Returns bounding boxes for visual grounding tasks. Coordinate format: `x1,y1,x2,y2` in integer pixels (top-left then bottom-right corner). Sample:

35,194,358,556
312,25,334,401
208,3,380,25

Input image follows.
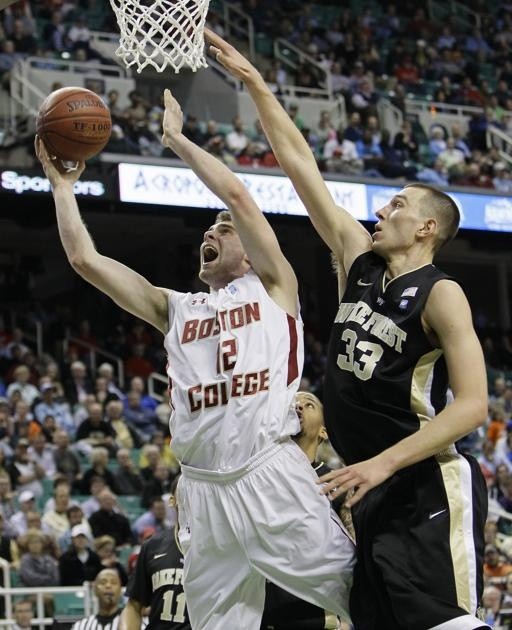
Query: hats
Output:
40,382,57,393
484,542,496,555
73,523,90,540
18,491,34,504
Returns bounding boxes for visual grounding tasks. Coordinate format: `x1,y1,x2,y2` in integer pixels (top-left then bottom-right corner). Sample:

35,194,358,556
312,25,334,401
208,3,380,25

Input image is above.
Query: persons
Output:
0,302,511,628
32,89,360,626
0,0,512,194
204,24,494,629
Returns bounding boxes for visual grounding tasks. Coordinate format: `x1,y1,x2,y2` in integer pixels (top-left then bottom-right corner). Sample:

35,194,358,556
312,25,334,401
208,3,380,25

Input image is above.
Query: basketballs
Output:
37,87,112,160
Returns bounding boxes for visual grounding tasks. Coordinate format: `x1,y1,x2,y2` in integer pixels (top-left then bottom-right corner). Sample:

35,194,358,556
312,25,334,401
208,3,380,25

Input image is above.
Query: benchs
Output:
0,424,155,629
